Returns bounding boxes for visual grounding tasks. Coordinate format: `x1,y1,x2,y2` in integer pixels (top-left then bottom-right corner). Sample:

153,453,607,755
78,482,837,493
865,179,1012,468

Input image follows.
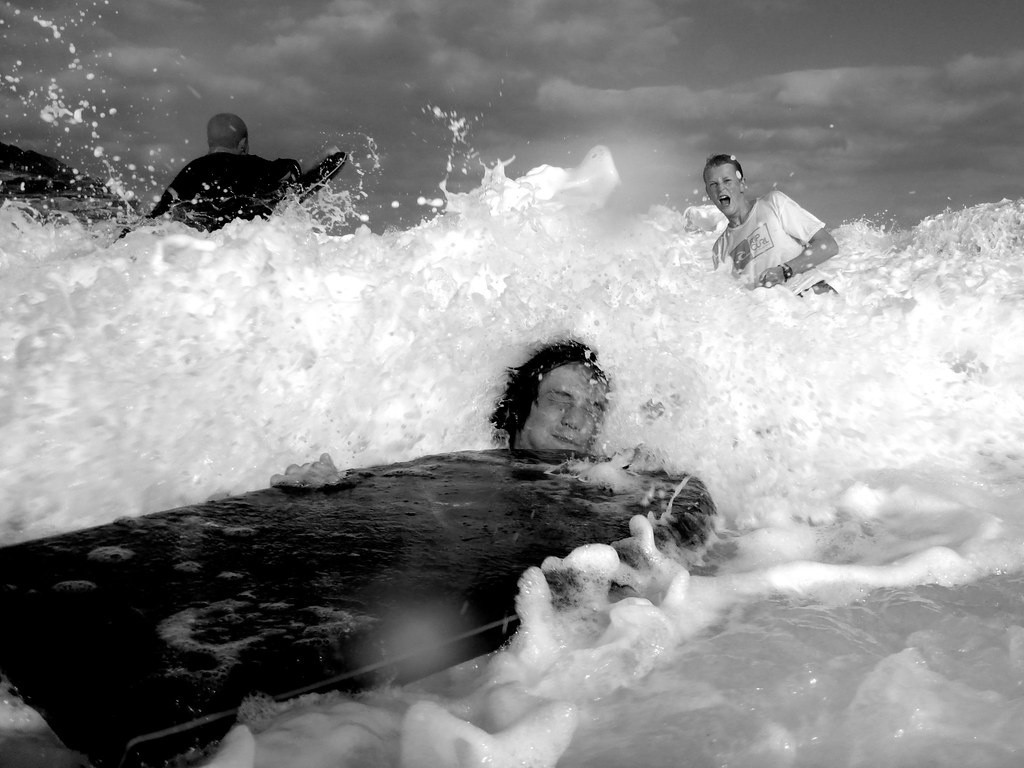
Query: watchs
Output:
779,264,793,281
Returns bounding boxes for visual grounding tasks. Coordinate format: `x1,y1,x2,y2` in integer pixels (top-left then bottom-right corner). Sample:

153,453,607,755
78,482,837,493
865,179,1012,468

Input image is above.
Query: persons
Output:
704,155,839,297
267,338,671,487
118,113,302,241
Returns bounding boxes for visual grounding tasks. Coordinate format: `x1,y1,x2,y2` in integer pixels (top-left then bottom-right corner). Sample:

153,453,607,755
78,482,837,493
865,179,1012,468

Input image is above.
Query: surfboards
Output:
286,151,347,204
0,443,715,768
